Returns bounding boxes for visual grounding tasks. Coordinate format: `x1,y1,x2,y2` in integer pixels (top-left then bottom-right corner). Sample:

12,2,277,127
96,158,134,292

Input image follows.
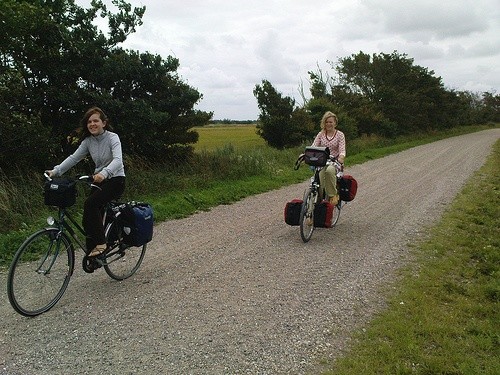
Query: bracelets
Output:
339,157,344,161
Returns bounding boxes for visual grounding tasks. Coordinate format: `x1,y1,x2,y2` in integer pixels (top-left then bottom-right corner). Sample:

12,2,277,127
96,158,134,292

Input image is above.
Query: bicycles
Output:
7,172,149,317
299,154,342,243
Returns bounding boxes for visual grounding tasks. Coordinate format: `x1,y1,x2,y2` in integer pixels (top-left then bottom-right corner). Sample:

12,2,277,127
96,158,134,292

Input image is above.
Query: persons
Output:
44,106,126,258
310,111,347,206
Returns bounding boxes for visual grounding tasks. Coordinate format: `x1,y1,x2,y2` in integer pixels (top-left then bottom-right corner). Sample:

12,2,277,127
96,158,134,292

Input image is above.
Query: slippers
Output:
88,242,109,257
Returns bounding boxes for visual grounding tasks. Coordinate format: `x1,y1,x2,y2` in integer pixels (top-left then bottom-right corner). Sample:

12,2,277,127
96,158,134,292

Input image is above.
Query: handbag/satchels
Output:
124,201,154,245
44,176,77,207
284,199,302,226
339,174,358,202
305,146,329,166
314,201,333,228
